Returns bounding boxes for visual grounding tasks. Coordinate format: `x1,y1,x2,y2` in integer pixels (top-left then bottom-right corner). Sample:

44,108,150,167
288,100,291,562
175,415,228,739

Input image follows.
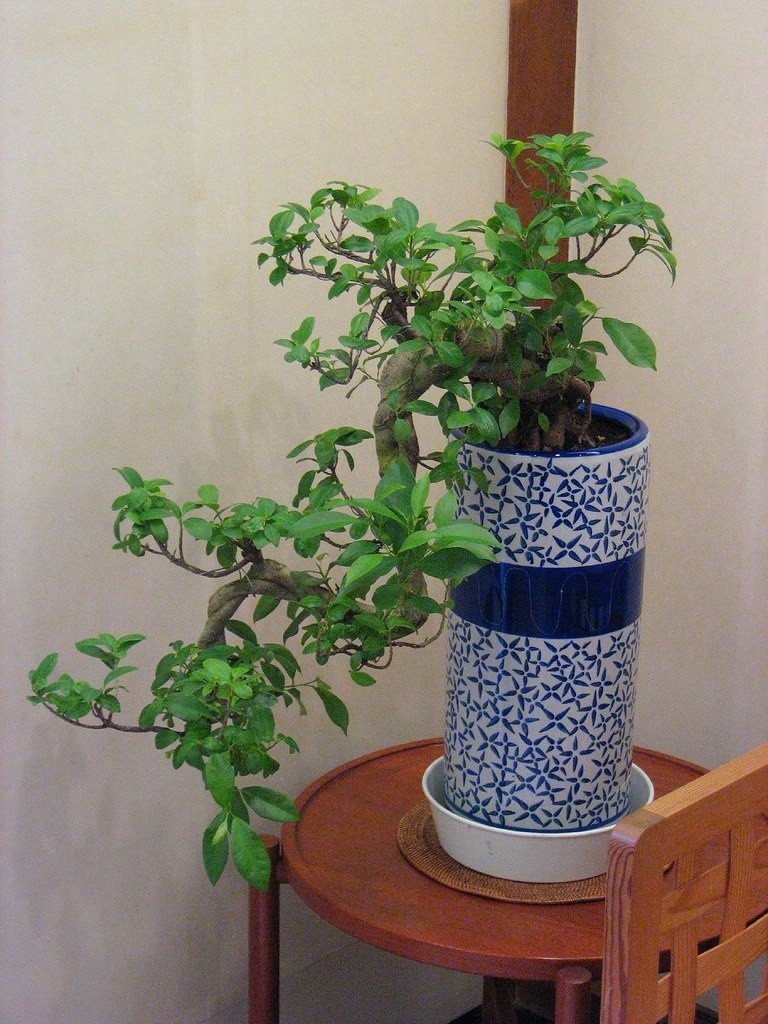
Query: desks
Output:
248,739,713,1024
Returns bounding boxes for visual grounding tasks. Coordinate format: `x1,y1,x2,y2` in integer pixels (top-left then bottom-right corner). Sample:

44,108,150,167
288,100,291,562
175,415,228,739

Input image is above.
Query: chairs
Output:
601,741,764,1023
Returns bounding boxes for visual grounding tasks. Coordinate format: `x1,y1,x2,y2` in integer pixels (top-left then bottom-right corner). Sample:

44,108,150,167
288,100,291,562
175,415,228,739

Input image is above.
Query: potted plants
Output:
24,128,677,893
